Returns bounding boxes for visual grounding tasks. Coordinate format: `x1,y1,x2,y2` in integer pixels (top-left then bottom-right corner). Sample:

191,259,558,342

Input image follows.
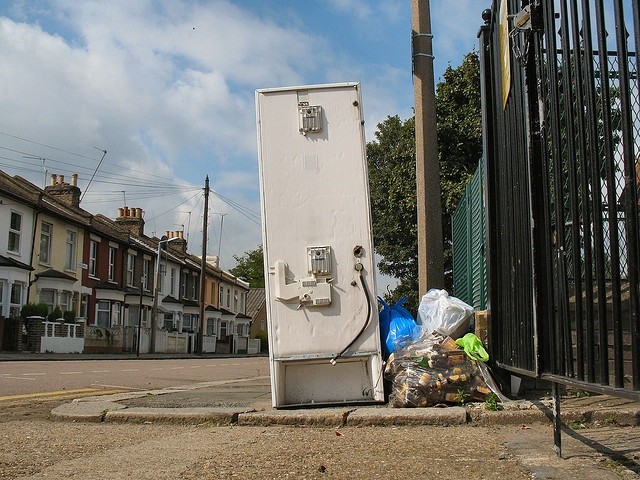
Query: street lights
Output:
152,237,180,301
